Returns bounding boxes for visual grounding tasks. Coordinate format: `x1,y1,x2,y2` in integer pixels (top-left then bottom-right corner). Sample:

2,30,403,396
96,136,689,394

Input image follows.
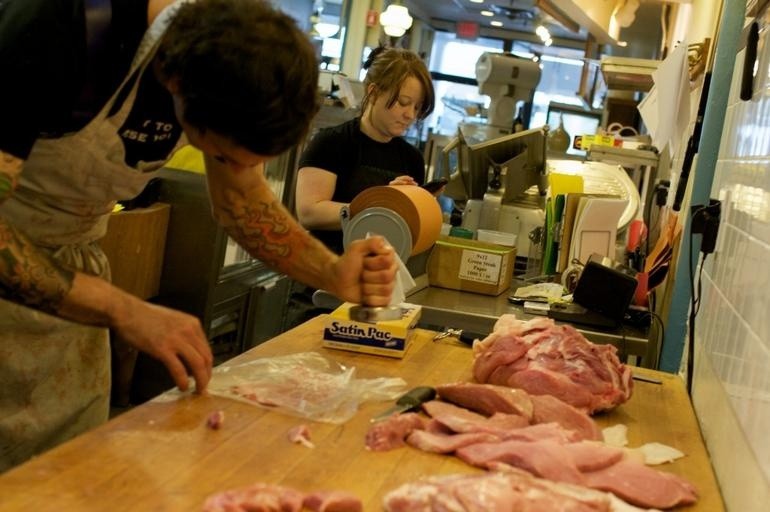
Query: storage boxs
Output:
431,231,518,298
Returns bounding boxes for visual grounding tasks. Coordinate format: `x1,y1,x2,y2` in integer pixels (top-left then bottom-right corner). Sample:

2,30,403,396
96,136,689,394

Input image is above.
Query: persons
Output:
1,1,400,473
293,43,443,328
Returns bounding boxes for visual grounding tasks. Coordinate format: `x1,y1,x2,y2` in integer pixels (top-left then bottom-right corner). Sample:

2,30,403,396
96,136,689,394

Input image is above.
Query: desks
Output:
401,285,653,357
2,312,734,512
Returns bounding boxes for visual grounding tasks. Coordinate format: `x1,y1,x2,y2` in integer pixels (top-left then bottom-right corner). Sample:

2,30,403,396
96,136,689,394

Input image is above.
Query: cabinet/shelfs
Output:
586,55,664,167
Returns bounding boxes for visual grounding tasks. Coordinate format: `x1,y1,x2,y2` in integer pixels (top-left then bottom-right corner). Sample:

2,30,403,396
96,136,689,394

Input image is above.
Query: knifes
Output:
369,385,436,423
